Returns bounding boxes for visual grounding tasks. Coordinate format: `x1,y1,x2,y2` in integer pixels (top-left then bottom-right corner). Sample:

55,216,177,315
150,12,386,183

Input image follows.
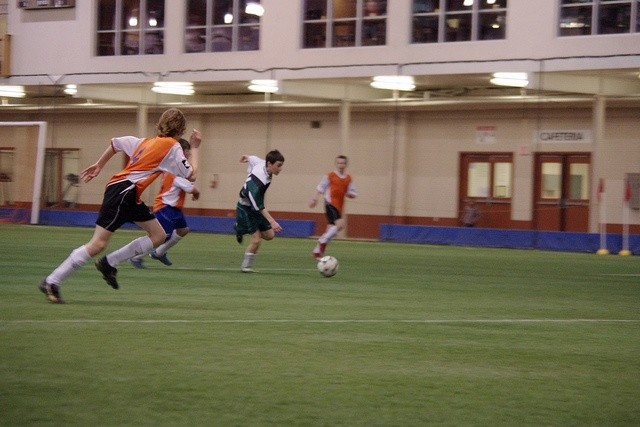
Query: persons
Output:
131,138,199,269
39,109,202,304
309,155,358,261
233,149,284,273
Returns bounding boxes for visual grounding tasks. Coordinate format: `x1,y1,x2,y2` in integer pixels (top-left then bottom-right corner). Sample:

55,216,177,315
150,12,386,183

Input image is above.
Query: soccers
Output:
317,256,339,277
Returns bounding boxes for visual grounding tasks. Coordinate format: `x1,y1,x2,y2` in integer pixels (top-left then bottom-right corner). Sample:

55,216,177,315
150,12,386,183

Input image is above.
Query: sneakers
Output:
128,258,147,269
240,267,260,273
233,222,242,243
94,255,119,289
149,250,172,265
38,278,62,303
312,250,320,260
318,239,327,256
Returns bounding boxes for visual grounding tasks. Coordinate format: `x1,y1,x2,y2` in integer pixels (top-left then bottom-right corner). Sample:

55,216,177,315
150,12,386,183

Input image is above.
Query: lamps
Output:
0,84,77,99
370,72,530,92
152,79,278,96
